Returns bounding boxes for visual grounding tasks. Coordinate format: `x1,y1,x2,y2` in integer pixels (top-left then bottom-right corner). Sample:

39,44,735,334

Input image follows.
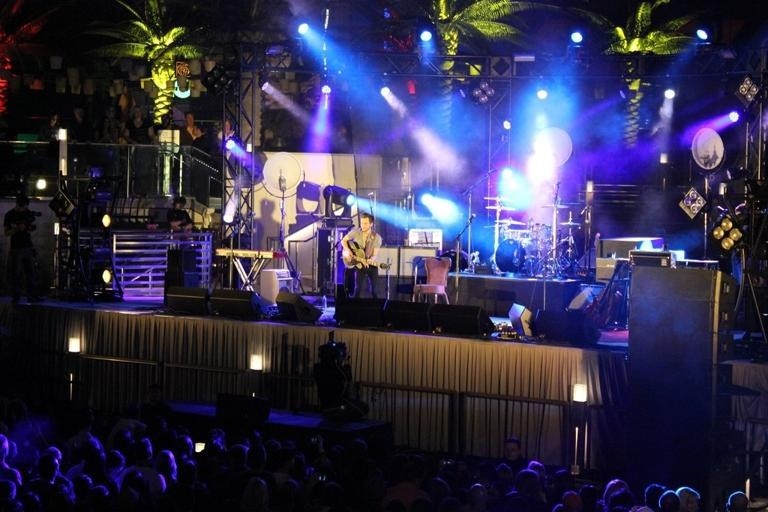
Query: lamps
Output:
573,383,588,425
323,184,353,227
249,353,264,391
67,338,81,368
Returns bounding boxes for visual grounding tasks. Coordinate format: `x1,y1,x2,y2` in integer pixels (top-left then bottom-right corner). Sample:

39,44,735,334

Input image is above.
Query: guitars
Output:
586,259,628,331
342,240,391,270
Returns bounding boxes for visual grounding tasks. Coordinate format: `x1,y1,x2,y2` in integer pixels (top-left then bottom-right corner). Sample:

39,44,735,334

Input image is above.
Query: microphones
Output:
467,213,478,224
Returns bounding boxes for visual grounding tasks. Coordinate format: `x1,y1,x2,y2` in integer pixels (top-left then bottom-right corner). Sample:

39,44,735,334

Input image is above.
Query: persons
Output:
3,192,45,306
0,420,764,512
167,196,193,231
49,67,372,204
341,214,382,299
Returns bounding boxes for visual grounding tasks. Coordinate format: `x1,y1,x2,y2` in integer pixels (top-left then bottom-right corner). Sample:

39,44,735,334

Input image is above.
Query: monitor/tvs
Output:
148,207,171,224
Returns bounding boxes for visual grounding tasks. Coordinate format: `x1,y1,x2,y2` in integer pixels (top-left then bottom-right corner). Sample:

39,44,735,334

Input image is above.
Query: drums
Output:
496,238,534,273
508,230,531,250
534,224,551,250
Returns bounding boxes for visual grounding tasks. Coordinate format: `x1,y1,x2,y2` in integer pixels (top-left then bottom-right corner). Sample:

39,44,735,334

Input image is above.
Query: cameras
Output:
13,210,42,226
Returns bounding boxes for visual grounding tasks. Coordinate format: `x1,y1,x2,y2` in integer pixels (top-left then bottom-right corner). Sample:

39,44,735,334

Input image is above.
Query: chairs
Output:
411,255,453,305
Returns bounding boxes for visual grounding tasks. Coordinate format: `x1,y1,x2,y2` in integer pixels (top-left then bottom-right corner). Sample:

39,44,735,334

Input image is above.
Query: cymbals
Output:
486,206,514,211
561,222,580,226
483,196,511,201
542,205,569,209
495,219,526,226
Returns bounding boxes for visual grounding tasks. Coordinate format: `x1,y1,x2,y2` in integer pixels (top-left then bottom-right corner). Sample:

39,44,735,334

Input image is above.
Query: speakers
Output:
274,290,323,323
335,297,496,339
531,308,603,346
622,265,739,511
165,286,268,321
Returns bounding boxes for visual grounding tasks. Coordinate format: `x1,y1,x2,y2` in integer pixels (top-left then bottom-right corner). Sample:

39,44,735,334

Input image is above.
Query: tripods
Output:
260,176,306,295
